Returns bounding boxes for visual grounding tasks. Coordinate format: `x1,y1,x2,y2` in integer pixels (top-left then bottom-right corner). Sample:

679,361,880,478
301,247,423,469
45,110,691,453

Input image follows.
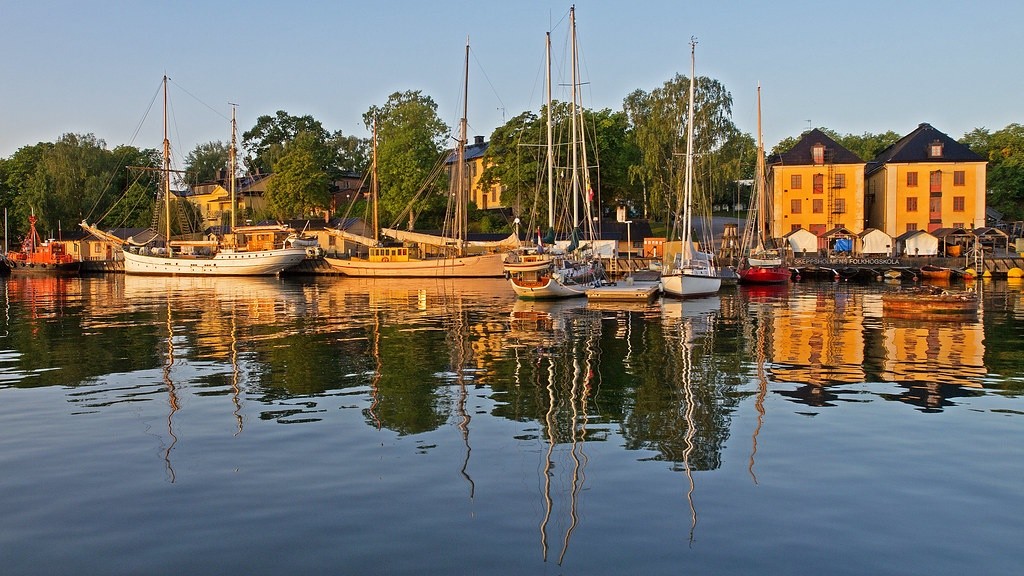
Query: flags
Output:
585,177,594,201
538,228,544,253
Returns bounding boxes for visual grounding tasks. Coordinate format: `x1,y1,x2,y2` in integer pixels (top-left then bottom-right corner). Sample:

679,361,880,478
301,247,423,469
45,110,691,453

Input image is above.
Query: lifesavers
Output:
3,259,46,268
381,257,389,262
306,246,319,258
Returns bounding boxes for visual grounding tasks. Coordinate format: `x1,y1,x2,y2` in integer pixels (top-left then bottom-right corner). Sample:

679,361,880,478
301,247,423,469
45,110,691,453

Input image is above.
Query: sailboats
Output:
76,4,790,304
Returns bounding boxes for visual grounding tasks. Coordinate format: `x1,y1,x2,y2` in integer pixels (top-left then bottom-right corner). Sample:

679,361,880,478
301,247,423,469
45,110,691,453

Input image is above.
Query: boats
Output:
7,207,79,268
920,265,951,279
